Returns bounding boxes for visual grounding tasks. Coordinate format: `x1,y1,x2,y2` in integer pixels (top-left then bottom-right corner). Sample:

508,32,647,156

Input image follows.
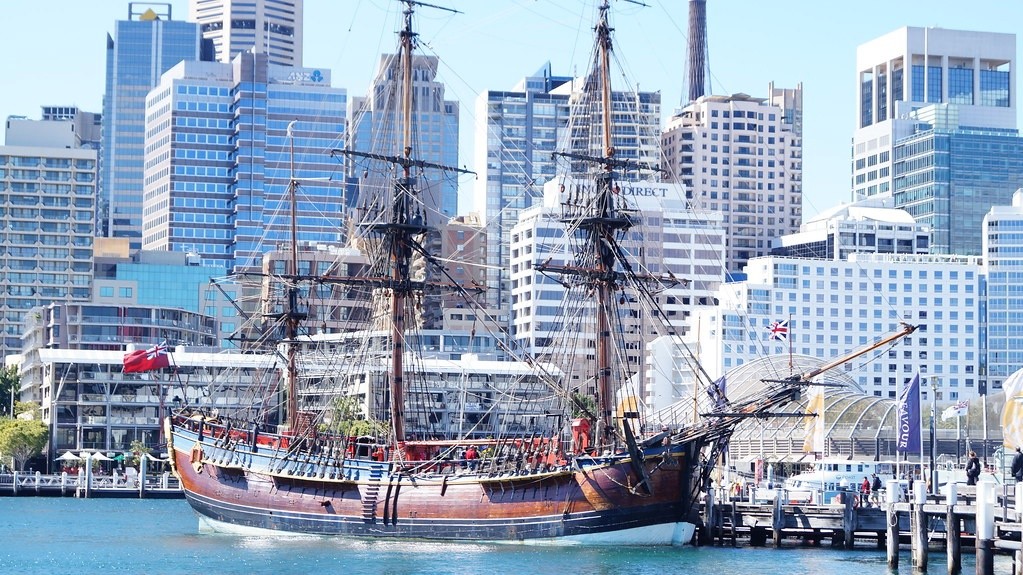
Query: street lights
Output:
923,374,947,492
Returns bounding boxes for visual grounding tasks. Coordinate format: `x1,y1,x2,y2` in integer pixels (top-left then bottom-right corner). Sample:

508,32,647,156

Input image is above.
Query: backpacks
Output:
967,462,980,477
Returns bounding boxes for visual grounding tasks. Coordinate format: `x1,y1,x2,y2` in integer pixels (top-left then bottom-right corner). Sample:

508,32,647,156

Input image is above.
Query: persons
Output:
170,412,698,484
861,471,919,509
716,474,788,506
0,464,15,482
1011,446,1023,482
63,461,172,477
963,450,981,504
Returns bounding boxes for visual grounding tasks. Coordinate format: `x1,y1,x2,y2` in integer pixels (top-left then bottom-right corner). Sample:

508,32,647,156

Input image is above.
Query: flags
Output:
123,341,170,374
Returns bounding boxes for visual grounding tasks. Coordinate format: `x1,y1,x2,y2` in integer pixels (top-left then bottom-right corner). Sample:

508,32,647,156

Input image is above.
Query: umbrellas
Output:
90,452,114,461
53,451,82,466
766,317,791,342
146,452,165,463
111,454,127,463
941,399,969,422
794,469,870,507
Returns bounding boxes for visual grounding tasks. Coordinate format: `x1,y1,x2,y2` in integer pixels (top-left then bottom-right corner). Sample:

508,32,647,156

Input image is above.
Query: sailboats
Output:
160,0,919,554
